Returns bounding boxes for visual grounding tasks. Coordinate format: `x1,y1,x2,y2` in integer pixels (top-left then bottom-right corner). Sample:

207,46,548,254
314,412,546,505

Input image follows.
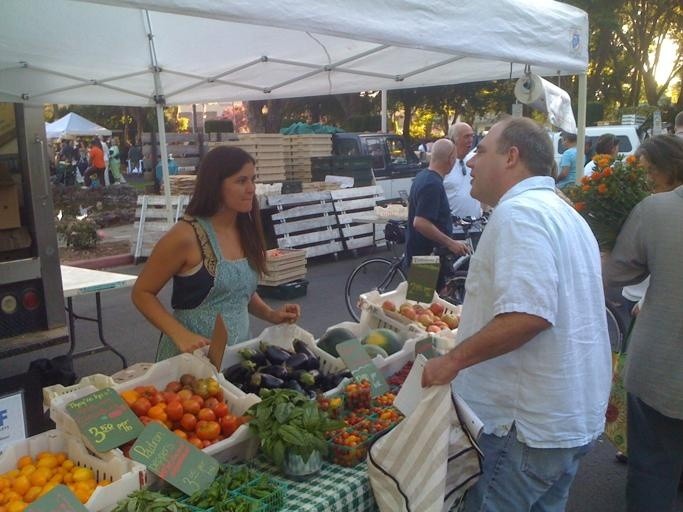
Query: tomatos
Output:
121,374,256,458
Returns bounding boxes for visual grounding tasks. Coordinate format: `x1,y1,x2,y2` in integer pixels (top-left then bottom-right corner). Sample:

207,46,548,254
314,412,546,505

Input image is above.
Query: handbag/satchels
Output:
366,383,484,512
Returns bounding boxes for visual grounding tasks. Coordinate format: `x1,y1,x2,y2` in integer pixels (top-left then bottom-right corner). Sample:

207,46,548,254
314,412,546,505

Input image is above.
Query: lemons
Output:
0,451,112,512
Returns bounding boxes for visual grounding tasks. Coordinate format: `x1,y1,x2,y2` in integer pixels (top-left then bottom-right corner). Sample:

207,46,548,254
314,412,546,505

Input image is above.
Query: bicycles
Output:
345,214,489,323
603,303,625,356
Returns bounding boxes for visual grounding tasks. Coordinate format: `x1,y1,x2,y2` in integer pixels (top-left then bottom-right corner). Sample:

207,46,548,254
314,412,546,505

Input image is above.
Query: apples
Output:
382,299,460,333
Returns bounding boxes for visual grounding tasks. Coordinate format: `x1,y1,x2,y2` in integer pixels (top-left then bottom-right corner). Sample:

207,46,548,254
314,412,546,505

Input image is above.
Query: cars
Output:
331,134,427,207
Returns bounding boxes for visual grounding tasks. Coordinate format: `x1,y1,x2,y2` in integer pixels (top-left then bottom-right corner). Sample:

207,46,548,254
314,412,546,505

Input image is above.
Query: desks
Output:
244,434,380,512
59,262,140,377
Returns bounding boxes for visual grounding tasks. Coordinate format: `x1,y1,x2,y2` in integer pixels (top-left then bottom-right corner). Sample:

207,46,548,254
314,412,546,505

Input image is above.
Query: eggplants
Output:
222,339,352,399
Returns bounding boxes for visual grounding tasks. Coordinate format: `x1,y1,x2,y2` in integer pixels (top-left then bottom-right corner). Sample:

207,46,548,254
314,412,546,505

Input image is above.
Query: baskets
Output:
315,321,423,380
48,352,256,487
359,281,462,339
320,403,405,468
0,428,147,512
151,462,288,512
193,322,355,403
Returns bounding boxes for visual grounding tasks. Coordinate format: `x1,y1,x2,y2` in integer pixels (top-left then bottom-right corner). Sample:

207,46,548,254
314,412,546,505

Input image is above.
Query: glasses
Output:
460,160,466,175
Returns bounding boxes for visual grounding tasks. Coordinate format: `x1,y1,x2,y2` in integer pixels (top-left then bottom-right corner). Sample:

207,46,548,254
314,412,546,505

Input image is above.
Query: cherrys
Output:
316,379,404,467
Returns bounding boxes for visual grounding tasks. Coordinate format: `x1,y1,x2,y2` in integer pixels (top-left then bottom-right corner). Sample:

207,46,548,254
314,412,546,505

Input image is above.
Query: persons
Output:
674,111,683,139
405,137,470,298
130,145,302,363
555,130,586,197
583,134,621,181
442,122,491,302
47,133,142,191
585,135,594,167
634,132,682,192
420,116,613,512
604,184,683,512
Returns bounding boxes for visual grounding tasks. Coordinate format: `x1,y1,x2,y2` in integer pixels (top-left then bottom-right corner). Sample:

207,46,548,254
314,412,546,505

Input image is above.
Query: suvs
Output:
547,124,641,173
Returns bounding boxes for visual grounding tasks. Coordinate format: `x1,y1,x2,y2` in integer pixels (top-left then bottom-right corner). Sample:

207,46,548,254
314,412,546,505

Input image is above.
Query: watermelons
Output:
316,328,404,359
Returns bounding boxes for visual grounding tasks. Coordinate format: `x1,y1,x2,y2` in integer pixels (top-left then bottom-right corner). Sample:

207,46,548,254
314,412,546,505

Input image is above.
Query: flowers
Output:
561,148,652,232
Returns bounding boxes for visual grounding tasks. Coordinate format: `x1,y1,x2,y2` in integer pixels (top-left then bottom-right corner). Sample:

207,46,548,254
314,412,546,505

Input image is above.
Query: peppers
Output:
186,463,281,512
